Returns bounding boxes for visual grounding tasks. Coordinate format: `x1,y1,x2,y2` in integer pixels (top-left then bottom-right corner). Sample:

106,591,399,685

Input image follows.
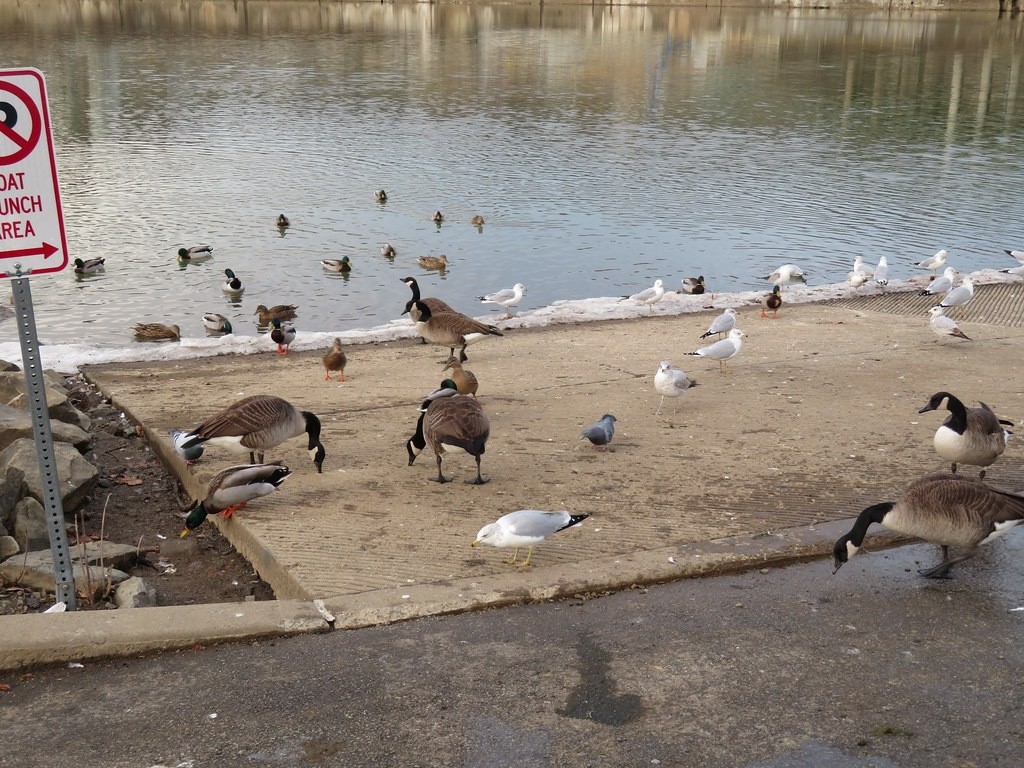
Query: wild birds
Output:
763,263,807,287
919,391,1014,481
910,248,1024,315
654,360,701,416
407,394,490,486
681,275,705,294
72,189,484,381
831,473,1023,578
475,282,528,318
846,255,890,293
682,329,749,374
180,459,294,539
472,510,593,569
926,306,973,345
180,394,327,474
758,284,782,319
700,307,740,342
399,277,504,364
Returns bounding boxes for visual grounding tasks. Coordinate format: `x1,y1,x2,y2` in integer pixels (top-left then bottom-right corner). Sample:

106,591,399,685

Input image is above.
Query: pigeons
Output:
167,428,208,466
580,414,617,452
